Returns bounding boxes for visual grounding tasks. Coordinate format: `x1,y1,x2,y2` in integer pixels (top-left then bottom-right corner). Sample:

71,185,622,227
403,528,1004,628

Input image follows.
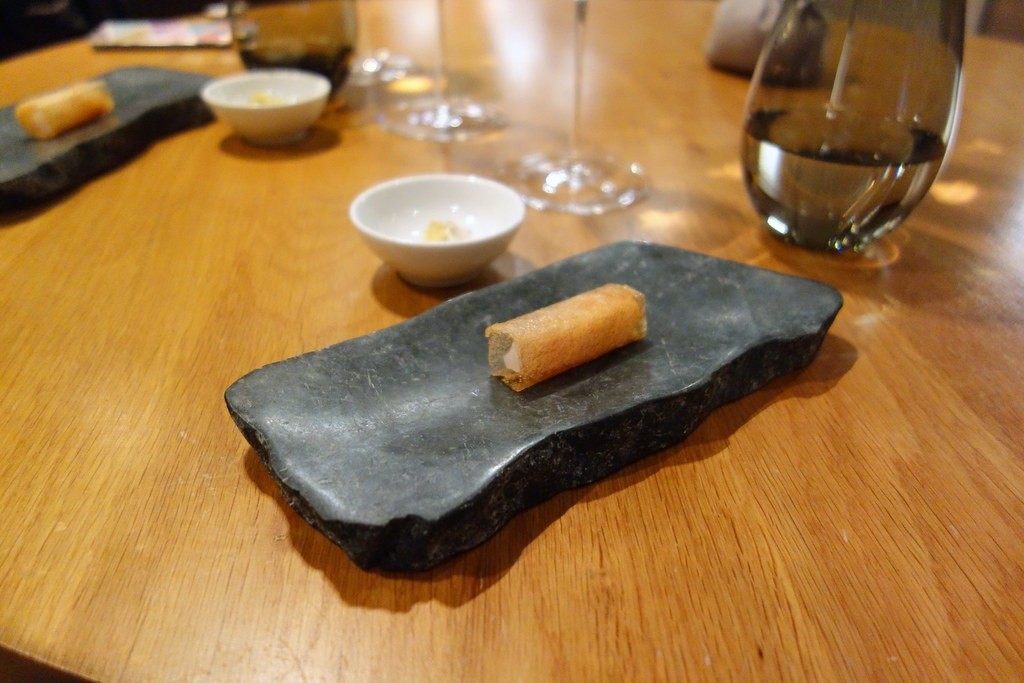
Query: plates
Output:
221,237,848,578
0,64,220,214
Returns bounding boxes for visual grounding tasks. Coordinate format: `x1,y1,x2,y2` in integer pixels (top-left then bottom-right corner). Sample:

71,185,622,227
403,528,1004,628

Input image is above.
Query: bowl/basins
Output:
353,174,528,292
202,71,331,148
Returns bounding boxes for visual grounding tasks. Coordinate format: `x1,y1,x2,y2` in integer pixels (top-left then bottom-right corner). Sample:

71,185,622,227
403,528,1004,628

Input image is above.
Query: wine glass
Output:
357,2,651,222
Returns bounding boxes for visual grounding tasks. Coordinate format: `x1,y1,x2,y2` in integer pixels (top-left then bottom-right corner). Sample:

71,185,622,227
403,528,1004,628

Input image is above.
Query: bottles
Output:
223,1,355,98
734,0,971,253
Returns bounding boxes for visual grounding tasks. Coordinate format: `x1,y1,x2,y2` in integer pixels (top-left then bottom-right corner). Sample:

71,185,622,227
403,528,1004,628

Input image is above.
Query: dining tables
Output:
0,0,1024,682
